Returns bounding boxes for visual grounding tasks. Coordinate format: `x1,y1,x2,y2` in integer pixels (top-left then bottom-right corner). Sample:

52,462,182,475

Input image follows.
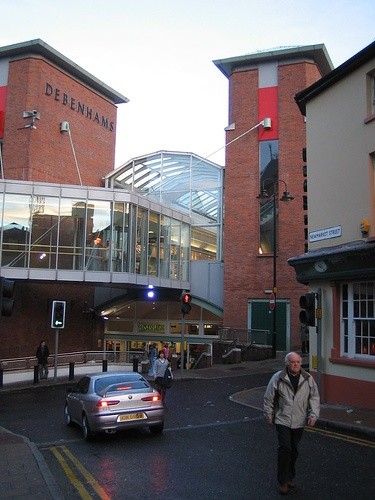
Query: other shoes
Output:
288,481,296,489
279,483,289,493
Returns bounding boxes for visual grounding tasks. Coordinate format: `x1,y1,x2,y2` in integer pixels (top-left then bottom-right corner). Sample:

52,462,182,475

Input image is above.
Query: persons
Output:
152,351,171,404
151,343,159,363
164,345,170,360
262,351,320,494
36,341,50,380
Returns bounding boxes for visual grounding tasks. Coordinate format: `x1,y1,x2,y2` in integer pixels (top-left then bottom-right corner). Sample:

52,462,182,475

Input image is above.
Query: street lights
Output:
256,178,294,359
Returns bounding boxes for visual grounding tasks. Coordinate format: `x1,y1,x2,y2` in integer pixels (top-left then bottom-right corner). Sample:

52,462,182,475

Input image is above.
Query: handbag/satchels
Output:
164,363,171,380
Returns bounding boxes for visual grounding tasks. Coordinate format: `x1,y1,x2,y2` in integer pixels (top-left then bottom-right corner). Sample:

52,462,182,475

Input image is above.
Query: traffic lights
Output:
180,293,192,313
51,301,65,329
298,292,316,327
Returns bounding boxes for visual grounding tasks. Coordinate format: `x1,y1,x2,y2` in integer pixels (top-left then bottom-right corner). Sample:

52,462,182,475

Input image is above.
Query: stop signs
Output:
269,293,276,310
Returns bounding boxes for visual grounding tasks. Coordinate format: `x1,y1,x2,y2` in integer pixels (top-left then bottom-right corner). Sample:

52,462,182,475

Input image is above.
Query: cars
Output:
63,372,165,445
